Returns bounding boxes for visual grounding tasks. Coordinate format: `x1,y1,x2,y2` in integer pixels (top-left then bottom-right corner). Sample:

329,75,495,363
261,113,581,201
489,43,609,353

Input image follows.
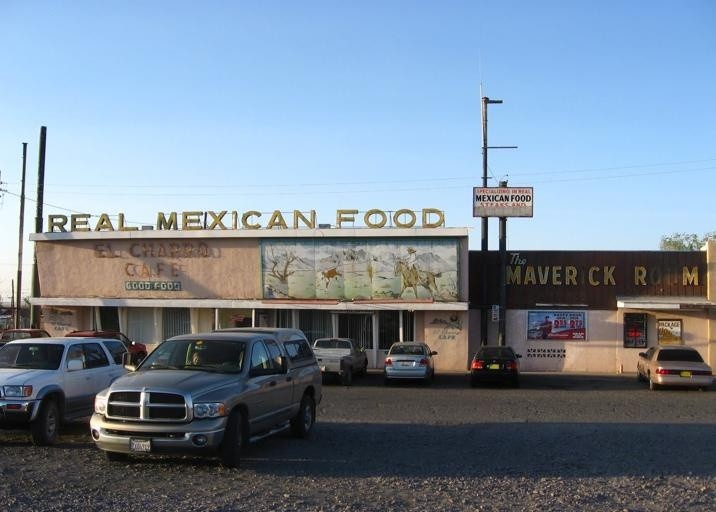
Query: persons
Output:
539,317,553,339
191,353,202,367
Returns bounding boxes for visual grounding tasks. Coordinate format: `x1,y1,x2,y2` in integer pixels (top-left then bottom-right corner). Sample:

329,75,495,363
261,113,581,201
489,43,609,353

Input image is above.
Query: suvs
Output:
0,335,148,448
66,329,148,367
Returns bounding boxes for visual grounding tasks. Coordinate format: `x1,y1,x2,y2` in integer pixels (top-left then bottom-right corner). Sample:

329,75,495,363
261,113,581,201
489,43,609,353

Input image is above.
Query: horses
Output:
393,259,442,303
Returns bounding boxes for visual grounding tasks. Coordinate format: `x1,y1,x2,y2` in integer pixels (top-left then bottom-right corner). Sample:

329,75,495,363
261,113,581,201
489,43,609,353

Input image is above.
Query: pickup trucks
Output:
310,336,369,385
88,323,324,470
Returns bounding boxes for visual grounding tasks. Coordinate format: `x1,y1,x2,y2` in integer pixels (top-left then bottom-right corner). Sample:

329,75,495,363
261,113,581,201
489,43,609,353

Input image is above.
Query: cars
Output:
469,342,524,390
380,340,440,387
0,326,52,352
634,345,715,391
